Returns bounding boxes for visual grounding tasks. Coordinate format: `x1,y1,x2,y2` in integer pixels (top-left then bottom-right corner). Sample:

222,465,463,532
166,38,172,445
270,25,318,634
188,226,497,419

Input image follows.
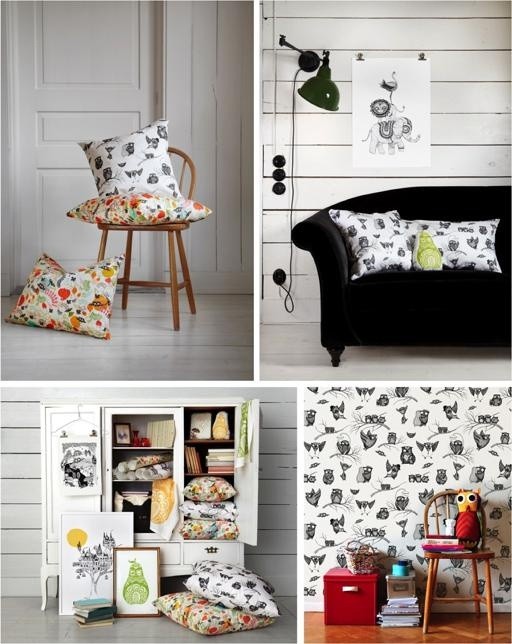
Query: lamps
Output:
276,31,340,112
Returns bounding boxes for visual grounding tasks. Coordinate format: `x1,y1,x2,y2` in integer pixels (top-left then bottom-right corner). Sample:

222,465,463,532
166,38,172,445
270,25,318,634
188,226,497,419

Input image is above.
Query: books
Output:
183,445,235,474
422,534,472,555
377,596,422,627
73,598,118,628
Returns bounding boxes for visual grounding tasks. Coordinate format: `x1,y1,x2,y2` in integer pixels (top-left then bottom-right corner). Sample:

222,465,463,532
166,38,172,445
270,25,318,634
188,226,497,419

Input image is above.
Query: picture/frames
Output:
59,511,165,617
114,421,132,447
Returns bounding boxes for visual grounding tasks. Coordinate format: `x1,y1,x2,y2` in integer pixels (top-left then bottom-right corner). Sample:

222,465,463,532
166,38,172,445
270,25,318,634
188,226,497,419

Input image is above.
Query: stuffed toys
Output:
452,488,482,550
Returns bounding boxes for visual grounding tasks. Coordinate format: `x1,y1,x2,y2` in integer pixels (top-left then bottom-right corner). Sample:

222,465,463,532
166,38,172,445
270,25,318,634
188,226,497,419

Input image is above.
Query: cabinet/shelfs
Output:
101,397,259,610
39,397,104,612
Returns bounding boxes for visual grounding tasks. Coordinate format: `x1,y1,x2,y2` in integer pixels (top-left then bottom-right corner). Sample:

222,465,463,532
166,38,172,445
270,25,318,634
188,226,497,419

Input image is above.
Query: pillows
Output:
178,475,240,539
66,117,214,225
325,205,503,279
5,252,126,339
154,559,281,634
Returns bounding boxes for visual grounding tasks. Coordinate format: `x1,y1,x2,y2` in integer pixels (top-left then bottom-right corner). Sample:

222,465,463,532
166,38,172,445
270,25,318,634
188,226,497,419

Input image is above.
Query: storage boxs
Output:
323,565,416,624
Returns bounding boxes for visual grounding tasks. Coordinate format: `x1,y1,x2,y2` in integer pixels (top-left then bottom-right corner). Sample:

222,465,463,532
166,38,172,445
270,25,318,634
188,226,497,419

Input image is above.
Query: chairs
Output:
422,489,495,634
97,147,197,330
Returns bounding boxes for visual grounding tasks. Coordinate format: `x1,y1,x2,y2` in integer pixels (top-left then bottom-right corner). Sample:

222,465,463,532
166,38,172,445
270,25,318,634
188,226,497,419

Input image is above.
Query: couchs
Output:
291,185,510,366
